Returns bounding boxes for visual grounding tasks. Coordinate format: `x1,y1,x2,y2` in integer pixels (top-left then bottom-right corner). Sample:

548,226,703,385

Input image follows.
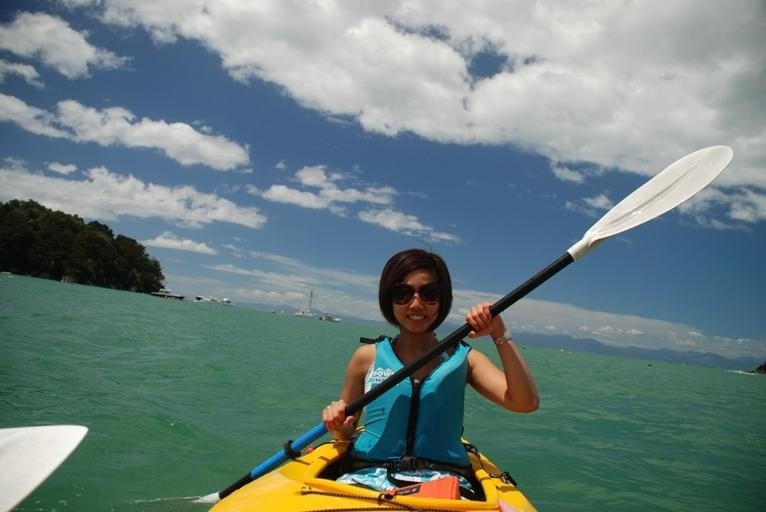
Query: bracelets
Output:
493,333,512,345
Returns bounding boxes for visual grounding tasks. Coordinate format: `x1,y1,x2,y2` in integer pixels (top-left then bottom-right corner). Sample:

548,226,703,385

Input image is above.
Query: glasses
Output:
391,283,441,304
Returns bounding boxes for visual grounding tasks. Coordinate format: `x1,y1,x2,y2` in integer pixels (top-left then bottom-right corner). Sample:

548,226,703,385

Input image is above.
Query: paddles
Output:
193,145,734,505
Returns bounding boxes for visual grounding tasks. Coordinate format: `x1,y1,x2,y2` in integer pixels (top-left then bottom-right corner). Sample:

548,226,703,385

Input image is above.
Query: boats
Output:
206,431,536,512
1,423,90,512
148,284,341,325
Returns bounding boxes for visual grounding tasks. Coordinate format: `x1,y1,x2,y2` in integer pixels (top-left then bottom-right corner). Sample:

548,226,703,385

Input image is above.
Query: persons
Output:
321,249,539,503
